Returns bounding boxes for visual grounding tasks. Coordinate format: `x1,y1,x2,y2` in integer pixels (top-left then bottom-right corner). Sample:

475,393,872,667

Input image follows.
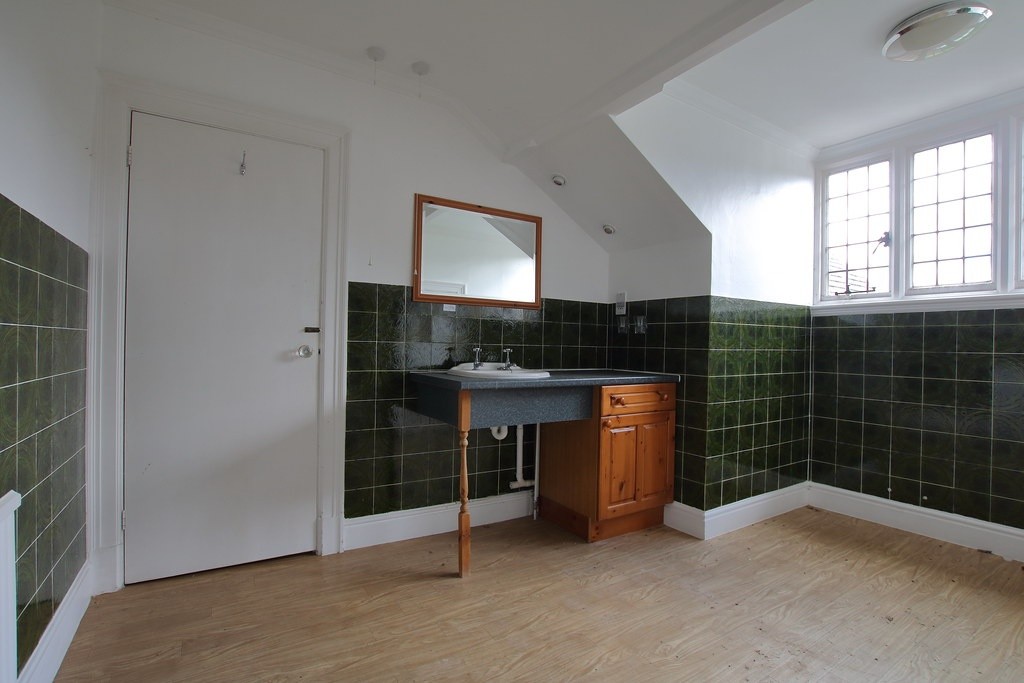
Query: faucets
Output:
472,348,485,370
498,349,516,370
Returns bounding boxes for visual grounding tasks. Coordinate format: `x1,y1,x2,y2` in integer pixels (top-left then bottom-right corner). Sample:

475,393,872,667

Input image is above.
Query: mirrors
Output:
412,193,541,310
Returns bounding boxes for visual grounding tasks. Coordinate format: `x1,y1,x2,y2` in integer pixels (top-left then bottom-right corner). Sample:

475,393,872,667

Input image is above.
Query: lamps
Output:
881,0,993,63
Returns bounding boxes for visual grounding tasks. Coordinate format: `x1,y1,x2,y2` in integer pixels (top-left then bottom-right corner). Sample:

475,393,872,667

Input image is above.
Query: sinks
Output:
447,360,550,380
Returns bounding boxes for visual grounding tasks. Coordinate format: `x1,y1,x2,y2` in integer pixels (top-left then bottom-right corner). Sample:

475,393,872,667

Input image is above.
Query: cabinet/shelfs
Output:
538,383,677,544
